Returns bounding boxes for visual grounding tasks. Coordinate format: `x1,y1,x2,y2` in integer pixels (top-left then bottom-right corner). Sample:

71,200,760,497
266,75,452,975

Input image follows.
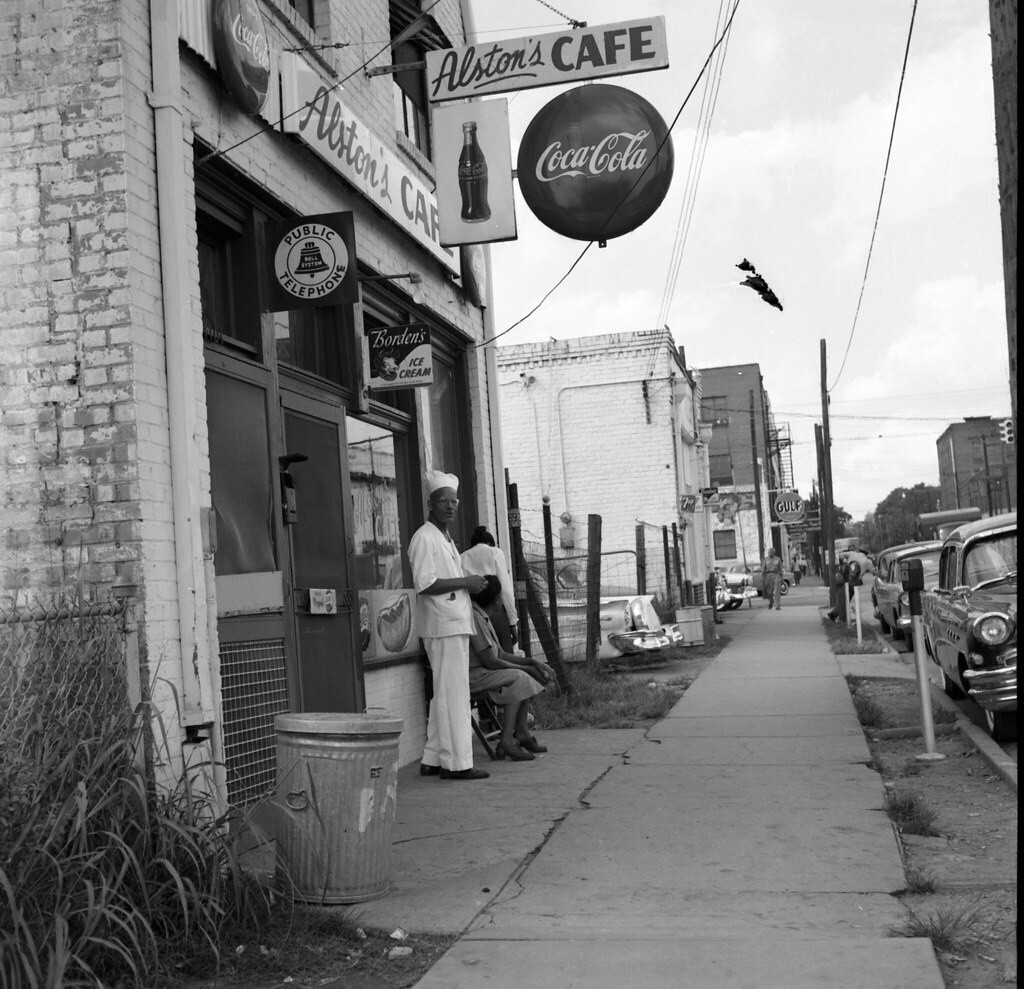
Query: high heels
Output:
519,737,548,753
495,741,535,760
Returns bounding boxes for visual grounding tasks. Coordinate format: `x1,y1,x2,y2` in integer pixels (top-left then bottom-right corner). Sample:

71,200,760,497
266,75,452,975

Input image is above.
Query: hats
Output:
424,469,459,496
467,573,503,607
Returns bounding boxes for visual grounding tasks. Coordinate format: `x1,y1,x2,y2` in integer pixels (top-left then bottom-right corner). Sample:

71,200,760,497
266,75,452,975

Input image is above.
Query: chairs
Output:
425,668,504,760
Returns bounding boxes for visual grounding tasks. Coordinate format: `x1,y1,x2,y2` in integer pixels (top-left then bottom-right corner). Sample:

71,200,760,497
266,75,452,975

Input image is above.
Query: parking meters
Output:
840,561,853,630
896,555,945,765
849,559,864,647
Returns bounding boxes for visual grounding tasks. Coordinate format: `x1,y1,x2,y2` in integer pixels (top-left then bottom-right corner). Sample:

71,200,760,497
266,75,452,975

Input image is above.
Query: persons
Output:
406,470,490,779
468,575,556,761
801,558,807,575
828,545,886,624
761,548,783,610
459,526,518,741
792,557,801,586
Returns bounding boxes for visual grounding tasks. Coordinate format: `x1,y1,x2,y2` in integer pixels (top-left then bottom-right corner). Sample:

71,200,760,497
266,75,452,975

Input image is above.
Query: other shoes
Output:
438,767,490,780
827,611,837,621
776,606,781,610
420,763,439,776
768,601,774,609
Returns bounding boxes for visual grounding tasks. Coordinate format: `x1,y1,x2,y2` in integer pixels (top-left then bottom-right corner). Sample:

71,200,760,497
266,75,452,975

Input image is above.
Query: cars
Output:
523,552,661,612
726,562,796,596
919,511,1018,739
869,539,943,652
526,577,683,663
714,567,754,612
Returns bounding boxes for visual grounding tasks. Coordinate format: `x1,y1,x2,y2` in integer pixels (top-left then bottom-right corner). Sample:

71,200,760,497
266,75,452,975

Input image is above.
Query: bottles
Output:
457,121,492,224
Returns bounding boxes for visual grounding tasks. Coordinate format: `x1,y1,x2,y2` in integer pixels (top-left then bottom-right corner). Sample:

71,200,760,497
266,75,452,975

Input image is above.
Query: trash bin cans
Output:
274,707,404,904
